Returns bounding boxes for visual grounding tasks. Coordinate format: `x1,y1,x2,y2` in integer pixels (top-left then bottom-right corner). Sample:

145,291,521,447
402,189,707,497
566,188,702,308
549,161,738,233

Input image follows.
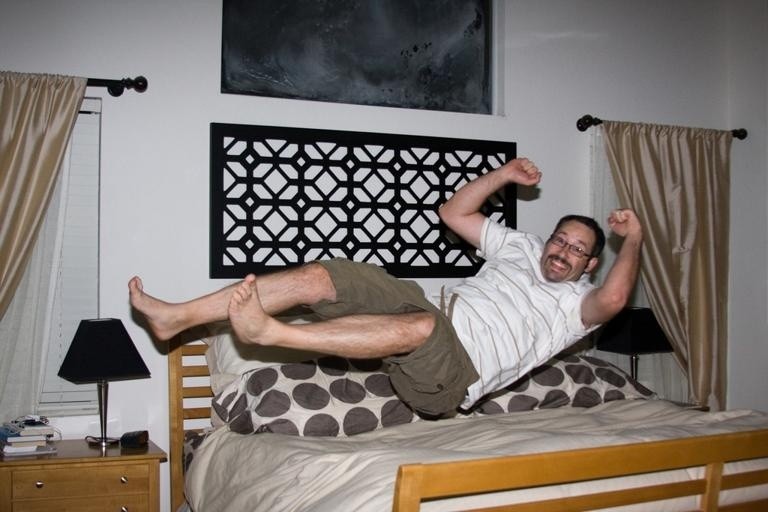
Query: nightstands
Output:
661,396,712,414
1,436,169,511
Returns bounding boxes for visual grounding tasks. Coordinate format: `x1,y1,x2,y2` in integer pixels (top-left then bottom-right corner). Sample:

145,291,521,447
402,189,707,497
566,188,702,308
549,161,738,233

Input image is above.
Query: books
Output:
0,421,59,457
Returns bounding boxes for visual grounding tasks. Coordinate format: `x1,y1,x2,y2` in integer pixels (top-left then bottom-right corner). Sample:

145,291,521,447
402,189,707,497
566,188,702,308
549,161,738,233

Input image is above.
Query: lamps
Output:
594,305,677,384
55,316,149,451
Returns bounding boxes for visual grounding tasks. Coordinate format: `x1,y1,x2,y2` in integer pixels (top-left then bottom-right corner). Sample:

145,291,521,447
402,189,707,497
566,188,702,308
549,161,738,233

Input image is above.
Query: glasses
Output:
551,234,591,257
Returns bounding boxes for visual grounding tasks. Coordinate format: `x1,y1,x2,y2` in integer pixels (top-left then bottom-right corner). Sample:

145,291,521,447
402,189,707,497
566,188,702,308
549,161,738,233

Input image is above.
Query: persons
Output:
127,157,645,420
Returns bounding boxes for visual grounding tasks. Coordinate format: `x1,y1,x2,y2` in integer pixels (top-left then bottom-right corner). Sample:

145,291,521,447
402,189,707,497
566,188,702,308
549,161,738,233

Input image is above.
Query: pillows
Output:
196,309,321,429
440,353,658,420
209,354,429,438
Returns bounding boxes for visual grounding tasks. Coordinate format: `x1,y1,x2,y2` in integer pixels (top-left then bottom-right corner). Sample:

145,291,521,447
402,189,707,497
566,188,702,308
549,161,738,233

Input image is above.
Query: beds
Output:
164,302,768,510
